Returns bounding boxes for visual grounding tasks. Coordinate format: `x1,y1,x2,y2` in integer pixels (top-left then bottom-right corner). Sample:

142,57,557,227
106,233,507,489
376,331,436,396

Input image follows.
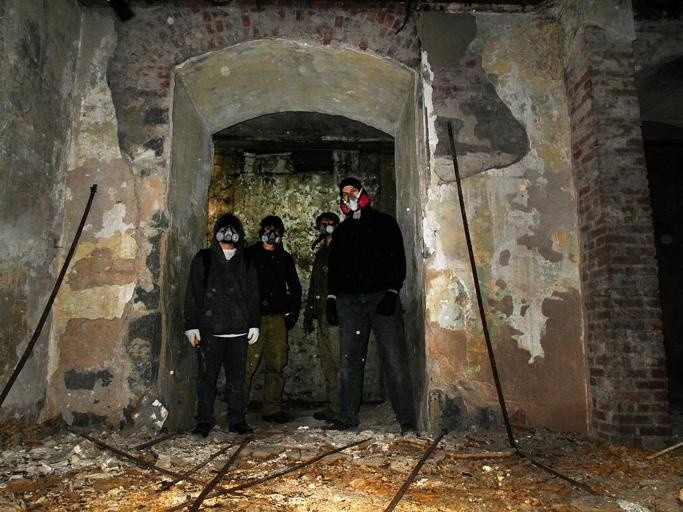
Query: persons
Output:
321,176,423,438
303,212,343,423
243,214,303,425
182,211,264,440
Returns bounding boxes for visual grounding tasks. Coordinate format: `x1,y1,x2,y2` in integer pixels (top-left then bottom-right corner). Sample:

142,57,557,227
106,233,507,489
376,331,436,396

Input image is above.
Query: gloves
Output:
286,313,299,330
184,328,202,348
303,317,316,333
247,328,260,345
376,291,398,316
325,298,339,326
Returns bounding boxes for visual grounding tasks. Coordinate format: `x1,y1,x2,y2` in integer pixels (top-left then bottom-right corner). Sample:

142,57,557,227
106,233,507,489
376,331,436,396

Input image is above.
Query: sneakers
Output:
191,407,420,439
